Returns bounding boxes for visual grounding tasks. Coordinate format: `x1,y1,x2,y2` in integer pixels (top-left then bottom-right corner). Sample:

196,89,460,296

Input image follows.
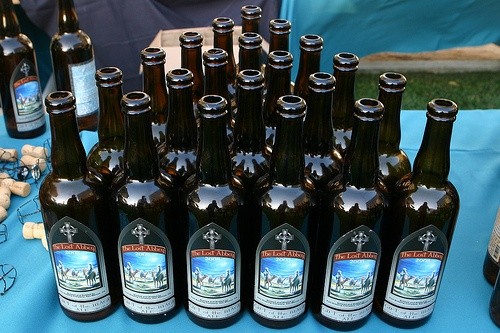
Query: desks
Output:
0,110,500,333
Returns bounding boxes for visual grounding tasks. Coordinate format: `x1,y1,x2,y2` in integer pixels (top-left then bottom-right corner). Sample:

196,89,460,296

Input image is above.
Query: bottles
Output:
482,205,500,287
488,269,500,330
0,0,461,331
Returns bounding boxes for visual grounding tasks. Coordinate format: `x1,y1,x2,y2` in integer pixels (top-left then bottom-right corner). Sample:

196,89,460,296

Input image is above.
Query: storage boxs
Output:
139,26,269,75
